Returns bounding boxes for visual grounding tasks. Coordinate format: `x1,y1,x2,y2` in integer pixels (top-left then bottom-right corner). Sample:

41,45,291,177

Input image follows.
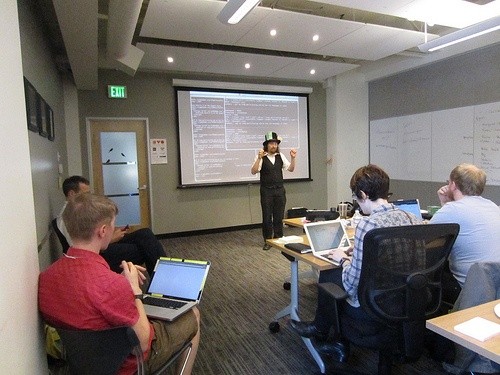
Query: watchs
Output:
340,258,349,265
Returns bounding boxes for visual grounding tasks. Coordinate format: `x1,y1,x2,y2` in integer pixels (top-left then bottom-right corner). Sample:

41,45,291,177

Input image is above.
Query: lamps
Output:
217,0,500,55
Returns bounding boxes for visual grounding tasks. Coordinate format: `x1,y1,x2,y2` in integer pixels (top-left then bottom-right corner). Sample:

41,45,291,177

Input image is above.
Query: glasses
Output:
352,193,360,201
446,178,453,184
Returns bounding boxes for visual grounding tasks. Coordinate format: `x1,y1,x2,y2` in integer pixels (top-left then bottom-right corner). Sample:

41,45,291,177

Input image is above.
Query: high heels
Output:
289,318,328,343
311,336,348,363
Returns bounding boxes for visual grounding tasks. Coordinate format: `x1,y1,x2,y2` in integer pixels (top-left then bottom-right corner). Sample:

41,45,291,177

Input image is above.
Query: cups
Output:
427,206,441,215
339,204,347,219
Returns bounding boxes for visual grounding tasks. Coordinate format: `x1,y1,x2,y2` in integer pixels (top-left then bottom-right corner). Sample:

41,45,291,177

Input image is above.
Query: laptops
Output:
304,220,353,266
392,199,422,221
141,257,210,322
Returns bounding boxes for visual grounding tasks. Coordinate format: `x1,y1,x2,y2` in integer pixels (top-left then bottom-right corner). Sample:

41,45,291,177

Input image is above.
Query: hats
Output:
263,131,281,146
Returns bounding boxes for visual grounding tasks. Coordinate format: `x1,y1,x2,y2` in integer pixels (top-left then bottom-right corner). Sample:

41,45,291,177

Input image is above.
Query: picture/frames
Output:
23,76,55,140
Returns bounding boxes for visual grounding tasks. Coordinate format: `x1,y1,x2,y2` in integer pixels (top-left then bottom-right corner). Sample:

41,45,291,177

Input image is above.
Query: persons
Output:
426,163,500,293
56,176,167,279
38,195,200,375
289,164,424,362
251,132,296,249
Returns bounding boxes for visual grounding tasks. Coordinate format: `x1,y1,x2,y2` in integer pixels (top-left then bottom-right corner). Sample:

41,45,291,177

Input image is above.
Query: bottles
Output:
351,210,362,228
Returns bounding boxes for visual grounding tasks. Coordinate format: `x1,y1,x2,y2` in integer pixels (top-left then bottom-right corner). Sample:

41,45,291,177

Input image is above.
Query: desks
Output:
265,208,500,375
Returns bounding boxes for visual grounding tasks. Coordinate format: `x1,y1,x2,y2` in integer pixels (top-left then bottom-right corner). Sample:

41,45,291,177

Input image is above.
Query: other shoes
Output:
263,244,271,250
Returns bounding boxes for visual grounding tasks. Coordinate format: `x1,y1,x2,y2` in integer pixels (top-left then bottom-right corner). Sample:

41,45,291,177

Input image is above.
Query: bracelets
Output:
258,157,261,159
134,295,144,301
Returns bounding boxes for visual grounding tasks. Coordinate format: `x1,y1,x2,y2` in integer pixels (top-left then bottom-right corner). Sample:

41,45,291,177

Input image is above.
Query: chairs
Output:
38,312,193,375
51,217,145,275
315,223,460,375
